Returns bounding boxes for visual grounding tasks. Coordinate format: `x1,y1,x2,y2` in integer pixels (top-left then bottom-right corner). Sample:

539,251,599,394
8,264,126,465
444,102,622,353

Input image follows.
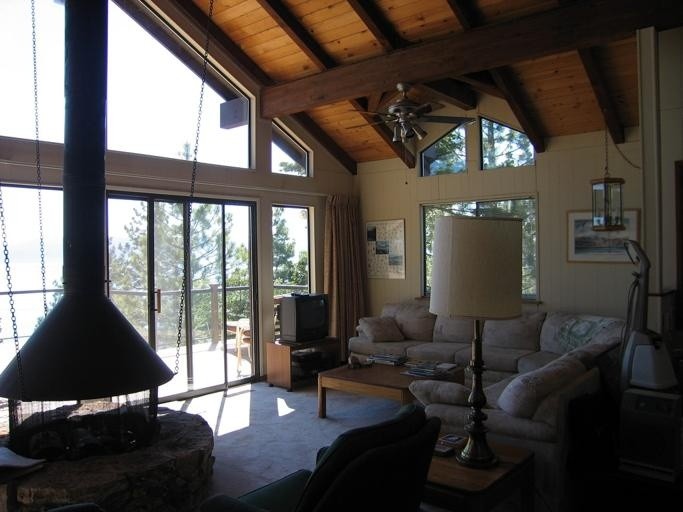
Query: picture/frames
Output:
567,209,641,264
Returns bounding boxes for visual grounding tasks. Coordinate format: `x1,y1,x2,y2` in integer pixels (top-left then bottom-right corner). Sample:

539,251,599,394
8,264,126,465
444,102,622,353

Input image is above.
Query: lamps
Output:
429,218,523,468
393,118,427,143
591,128,626,230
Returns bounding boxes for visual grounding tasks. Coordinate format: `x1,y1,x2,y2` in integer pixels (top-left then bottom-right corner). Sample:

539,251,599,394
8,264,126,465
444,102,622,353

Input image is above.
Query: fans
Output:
346,82,475,131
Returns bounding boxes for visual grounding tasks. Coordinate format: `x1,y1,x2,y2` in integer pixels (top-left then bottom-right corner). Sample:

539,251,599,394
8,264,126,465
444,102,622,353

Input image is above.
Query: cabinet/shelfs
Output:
267,339,339,390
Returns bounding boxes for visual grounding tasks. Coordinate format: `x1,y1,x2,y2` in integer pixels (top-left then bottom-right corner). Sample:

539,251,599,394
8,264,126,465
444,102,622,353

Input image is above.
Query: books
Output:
366,351,408,366
401,357,457,378
435,432,467,458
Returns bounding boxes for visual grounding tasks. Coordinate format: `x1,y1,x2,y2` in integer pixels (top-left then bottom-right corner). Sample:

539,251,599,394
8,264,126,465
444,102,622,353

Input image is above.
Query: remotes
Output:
290,292,310,297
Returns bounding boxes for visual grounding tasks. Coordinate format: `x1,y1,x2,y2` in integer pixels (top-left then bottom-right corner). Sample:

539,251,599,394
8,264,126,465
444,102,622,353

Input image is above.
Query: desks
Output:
423,431,534,512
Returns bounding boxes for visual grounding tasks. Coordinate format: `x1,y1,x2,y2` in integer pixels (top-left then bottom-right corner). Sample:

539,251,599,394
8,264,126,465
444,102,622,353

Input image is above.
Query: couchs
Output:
199,404,442,512
348,301,624,511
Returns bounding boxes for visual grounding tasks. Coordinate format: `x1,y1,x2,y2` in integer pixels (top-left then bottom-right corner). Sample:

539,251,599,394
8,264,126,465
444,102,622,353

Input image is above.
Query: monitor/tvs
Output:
279,293,329,343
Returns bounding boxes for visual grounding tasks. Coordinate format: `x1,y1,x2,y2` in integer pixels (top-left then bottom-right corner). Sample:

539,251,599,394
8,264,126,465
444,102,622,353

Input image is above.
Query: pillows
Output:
360,317,403,342
409,380,492,407
